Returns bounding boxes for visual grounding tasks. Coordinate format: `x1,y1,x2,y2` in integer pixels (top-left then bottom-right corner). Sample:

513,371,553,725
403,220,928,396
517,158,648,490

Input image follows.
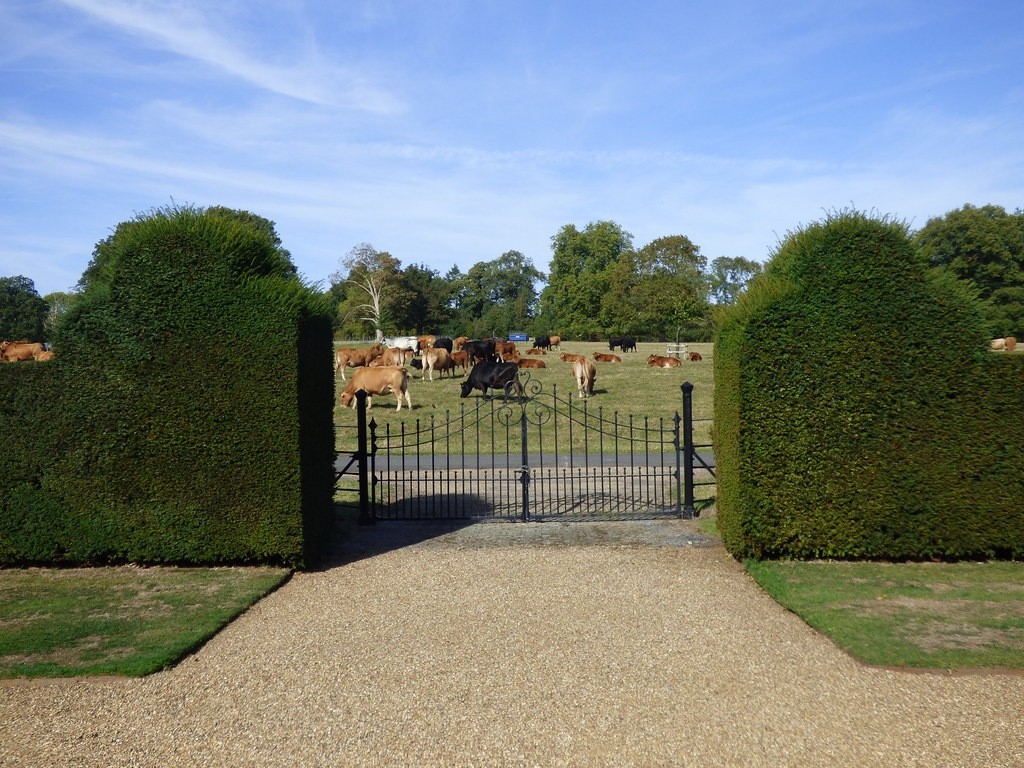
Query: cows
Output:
572,357,597,398
990,337,1017,352
335,335,703,383
460,361,522,400
338,366,412,411
0,341,55,362
